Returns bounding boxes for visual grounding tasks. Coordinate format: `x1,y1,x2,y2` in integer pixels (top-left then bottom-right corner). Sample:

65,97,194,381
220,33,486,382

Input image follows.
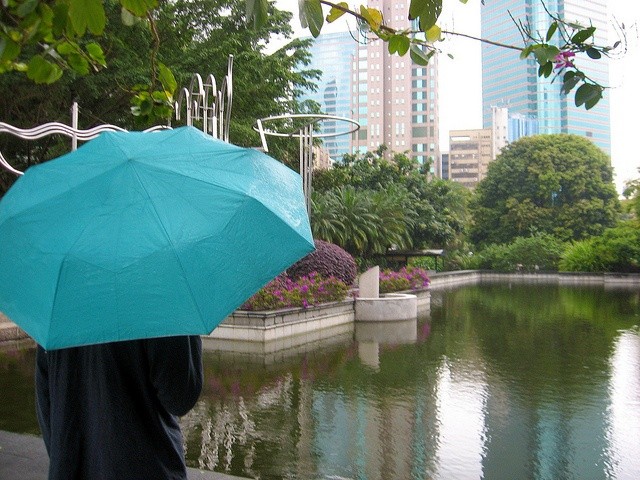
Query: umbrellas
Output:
0,125,320,355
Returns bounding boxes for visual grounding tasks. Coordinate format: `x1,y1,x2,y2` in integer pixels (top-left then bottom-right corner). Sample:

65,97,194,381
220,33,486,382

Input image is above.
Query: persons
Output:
34,333,204,479
